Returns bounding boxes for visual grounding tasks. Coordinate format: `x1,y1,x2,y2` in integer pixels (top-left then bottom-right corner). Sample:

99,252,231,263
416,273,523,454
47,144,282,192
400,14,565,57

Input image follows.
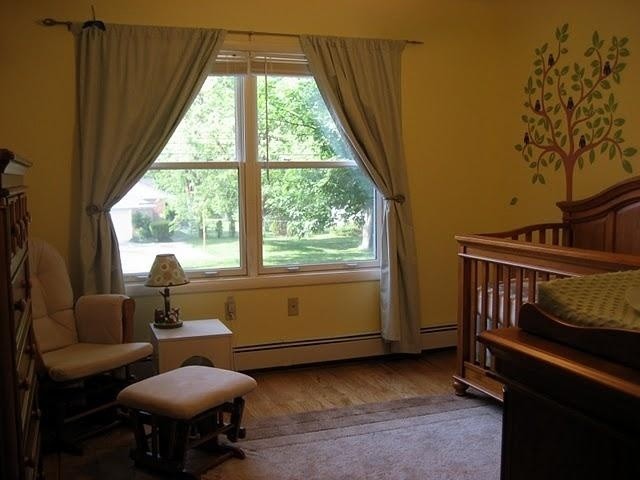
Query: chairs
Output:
25,227,156,465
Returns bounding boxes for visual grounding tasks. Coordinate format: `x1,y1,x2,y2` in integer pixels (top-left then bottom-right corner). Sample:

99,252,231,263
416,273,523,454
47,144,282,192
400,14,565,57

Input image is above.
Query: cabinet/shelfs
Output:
477,320,639,479
0,138,45,479
147,316,236,376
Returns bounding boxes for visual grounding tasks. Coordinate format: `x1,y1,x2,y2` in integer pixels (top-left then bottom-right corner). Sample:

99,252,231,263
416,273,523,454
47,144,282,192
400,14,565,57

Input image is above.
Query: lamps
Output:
145,251,187,329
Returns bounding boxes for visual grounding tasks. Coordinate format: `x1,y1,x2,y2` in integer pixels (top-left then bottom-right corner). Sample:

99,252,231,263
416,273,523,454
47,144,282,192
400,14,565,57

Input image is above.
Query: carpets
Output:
40,384,506,479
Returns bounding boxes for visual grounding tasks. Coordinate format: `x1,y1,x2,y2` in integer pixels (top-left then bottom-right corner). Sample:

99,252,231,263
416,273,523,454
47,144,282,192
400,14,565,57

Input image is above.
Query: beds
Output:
449,169,637,409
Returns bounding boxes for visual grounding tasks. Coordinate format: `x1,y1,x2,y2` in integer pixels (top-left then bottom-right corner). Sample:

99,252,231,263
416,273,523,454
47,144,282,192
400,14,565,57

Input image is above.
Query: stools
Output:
115,361,256,479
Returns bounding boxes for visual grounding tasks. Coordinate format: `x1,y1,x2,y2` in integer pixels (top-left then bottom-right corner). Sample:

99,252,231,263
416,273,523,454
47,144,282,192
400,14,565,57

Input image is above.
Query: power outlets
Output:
224,301,237,322
